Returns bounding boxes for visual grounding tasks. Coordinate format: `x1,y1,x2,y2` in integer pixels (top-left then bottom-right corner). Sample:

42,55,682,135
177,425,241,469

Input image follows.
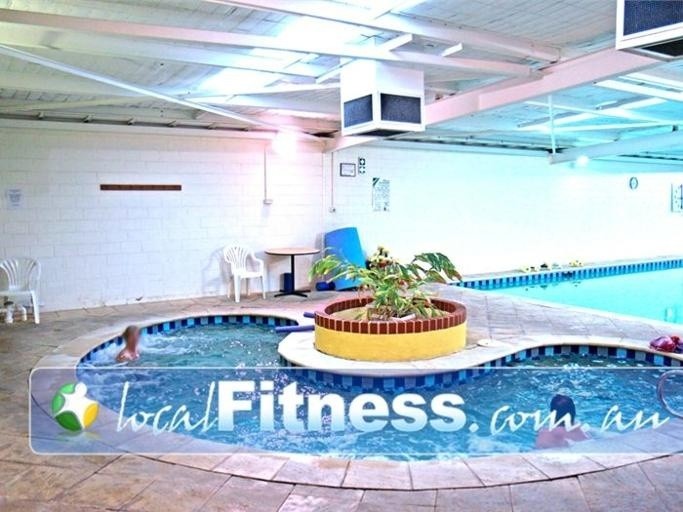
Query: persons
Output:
114,324,144,363
534,392,592,450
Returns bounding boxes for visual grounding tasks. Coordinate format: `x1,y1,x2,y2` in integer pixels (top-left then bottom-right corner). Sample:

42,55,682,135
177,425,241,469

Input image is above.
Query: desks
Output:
265,248,321,298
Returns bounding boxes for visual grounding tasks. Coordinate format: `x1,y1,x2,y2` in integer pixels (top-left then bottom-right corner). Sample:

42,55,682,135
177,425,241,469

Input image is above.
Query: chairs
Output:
222,244,266,302
0,256,42,324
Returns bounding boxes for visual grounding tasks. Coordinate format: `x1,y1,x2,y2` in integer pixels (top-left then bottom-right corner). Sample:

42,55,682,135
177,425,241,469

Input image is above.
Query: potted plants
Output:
309,242,467,362
